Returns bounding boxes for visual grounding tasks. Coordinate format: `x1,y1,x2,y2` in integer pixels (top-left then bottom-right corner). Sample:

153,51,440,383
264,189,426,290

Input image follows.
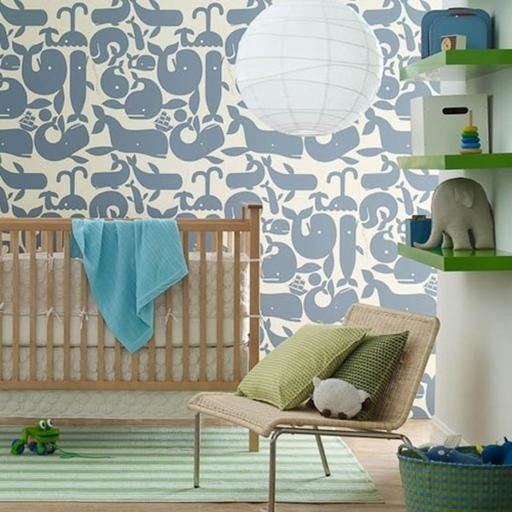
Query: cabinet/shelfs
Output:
397,49,512,274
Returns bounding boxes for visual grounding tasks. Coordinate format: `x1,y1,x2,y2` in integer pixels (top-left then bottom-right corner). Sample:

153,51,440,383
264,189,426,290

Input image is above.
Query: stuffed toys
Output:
310,376,372,420
424,436,512,465
412,177,495,252
10,417,61,455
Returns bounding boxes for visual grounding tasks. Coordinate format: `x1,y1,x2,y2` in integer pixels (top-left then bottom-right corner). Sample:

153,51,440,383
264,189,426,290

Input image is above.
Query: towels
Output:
70,219,188,354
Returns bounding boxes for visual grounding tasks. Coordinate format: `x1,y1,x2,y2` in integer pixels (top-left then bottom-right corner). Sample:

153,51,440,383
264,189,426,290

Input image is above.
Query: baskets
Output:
396,444,512,512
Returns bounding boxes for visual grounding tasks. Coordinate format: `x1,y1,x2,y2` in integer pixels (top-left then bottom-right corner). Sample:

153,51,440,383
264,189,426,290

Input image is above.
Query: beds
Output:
0,203,264,452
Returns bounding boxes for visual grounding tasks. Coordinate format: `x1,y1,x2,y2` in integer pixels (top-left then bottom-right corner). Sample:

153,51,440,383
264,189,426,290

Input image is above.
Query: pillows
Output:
332,329,409,420
237,323,373,411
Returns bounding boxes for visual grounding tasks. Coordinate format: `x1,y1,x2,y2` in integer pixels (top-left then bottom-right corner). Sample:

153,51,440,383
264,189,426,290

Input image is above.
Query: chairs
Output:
188,303,440,512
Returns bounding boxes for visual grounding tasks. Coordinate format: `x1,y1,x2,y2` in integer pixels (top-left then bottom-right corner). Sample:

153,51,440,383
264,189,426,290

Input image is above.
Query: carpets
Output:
0,420,385,502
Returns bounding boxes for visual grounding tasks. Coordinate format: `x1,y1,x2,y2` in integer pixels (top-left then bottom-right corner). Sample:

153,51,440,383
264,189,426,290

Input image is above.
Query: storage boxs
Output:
410,94,489,156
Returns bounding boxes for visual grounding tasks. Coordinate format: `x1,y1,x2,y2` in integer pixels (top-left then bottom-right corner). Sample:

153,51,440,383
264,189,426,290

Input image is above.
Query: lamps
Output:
235,0,384,136
